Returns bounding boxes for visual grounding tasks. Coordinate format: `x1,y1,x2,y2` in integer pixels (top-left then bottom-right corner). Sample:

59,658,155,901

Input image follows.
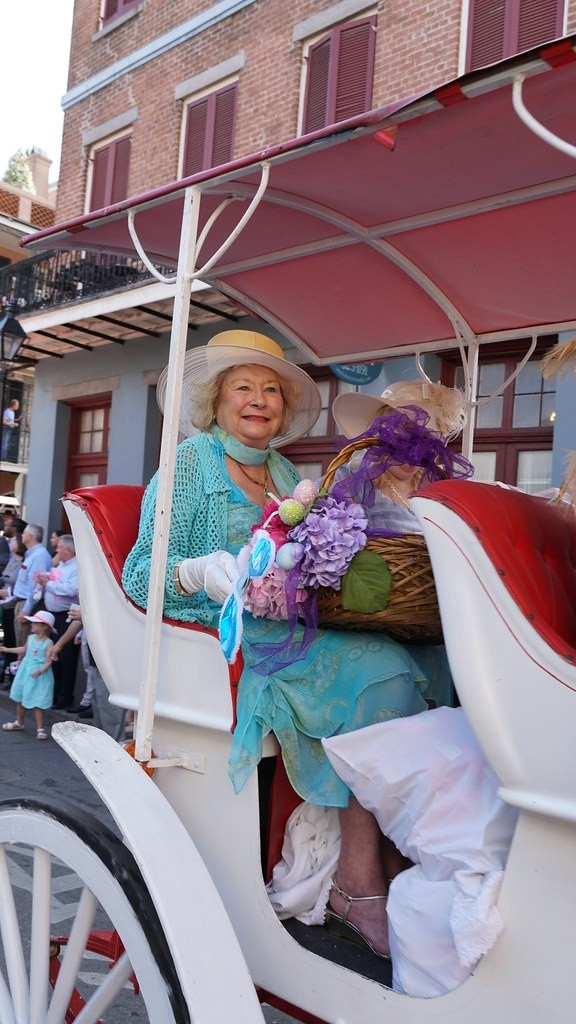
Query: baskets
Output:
296,438,450,646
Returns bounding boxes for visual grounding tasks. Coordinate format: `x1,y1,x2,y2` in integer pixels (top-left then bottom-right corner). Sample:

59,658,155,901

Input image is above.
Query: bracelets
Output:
173,561,192,596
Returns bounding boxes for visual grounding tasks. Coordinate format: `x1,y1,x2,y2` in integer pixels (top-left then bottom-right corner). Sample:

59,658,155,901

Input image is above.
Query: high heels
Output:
325,875,394,963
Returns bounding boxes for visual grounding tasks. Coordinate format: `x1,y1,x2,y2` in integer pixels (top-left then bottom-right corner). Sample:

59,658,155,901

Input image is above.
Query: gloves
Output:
179,550,240,604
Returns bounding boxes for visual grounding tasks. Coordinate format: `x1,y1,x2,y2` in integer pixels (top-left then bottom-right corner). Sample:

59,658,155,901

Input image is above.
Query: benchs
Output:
405,480,573,823
59,484,305,893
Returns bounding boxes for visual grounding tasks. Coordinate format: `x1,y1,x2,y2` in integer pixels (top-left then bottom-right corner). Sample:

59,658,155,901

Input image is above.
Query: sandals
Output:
2,721,24,731
36,729,47,740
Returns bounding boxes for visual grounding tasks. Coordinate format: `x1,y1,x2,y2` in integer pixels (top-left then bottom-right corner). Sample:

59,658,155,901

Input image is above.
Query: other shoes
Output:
52,702,73,710
79,708,93,718
66,704,90,713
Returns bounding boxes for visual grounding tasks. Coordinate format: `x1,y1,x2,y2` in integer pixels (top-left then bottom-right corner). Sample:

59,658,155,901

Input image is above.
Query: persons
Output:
121,330,430,958
1,399,23,460
0,513,134,742
0,610,54,740
316,381,467,534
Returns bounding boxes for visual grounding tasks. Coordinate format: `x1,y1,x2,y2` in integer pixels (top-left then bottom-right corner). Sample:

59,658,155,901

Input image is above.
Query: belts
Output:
16,598,26,601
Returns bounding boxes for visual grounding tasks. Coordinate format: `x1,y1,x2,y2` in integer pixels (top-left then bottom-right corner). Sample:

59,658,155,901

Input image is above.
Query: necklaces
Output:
386,479,411,509
33,638,46,656
237,461,270,498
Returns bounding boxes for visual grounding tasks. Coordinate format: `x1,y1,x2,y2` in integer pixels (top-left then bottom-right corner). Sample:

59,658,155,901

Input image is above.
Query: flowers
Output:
238,482,393,625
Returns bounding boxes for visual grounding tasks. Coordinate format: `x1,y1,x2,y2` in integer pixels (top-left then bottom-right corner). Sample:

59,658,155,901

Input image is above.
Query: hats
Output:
24,610,59,636
0,510,16,516
332,381,468,449
156,329,322,449
